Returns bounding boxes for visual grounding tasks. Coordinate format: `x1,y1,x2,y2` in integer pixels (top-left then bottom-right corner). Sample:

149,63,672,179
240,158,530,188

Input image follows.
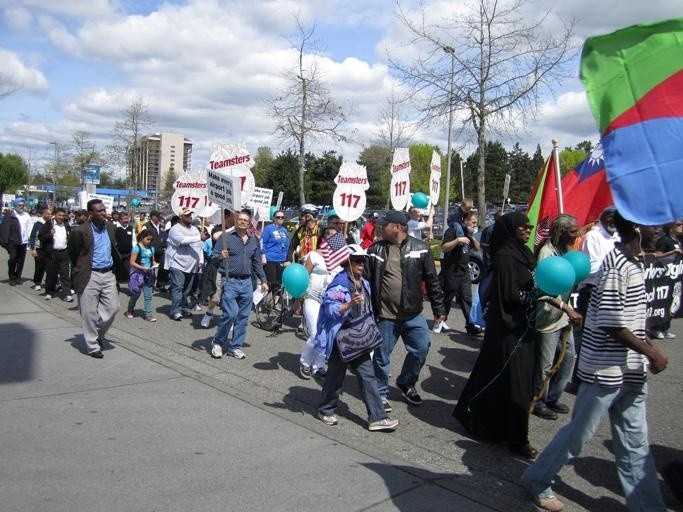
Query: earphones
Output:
634,226,641,235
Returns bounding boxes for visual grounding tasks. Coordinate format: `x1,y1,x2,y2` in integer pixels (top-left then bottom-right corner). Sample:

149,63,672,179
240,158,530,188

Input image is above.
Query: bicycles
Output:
253,278,313,337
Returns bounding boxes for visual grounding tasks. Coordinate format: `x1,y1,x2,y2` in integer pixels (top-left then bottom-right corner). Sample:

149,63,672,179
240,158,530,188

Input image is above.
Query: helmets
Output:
300,204,319,218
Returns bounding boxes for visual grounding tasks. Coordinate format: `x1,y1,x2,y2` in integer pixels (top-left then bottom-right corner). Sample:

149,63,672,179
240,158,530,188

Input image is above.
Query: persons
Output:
0,196,289,359
640,224,682,339
282,199,504,431
516,207,666,512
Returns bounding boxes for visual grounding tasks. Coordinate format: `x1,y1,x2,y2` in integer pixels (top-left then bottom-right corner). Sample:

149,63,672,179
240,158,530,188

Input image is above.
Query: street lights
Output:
441,43,455,239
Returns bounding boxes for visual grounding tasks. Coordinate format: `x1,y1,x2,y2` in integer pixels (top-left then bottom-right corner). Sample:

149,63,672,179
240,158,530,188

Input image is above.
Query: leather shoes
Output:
90,351,104,358
515,441,537,458
533,400,569,420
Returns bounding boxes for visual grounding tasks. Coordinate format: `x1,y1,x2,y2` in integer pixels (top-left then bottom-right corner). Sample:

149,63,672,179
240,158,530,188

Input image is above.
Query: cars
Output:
285,200,531,284
110,196,174,216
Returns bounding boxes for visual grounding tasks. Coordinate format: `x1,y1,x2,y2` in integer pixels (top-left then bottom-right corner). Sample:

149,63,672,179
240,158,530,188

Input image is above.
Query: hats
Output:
347,244,368,256
179,207,194,215
150,211,160,218
13,198,25,206
377,211,406,225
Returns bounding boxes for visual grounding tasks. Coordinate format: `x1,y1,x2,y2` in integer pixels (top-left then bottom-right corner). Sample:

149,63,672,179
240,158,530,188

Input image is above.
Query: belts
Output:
221,274,250,279
54,249,65,252
92,267,112,273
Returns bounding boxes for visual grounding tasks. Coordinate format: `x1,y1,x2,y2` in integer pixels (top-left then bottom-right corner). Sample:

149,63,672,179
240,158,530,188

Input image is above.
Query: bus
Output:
14,189,59,203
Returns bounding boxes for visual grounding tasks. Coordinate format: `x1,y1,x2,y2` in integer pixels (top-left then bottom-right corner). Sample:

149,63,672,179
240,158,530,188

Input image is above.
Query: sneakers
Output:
652,330,676,339
35,284,75,302
369,396,398,431
127,311,157,322
396,382,422,404
201,311,212,327
211,340,246,359
318,412,337,425
299,362,312,379
173,313,184,320
527,492,564,512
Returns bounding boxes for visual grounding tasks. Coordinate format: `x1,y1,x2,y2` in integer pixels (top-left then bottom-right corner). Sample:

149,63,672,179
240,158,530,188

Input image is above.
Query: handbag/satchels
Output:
336,312,383,363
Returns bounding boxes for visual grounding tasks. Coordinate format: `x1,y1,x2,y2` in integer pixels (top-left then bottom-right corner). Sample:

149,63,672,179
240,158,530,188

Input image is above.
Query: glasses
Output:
276,217,285,219
351,258,366,264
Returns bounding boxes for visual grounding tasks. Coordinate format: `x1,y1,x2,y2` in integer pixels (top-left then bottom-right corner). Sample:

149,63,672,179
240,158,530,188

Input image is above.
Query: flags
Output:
579,16,682,225
523,149,561,259
561,141,614,232
